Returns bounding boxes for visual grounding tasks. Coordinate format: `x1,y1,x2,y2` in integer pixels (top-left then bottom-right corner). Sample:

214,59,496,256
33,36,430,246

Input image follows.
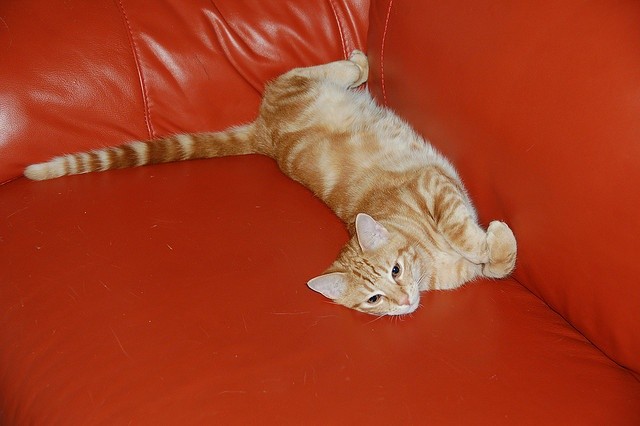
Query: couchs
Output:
0,0,639,426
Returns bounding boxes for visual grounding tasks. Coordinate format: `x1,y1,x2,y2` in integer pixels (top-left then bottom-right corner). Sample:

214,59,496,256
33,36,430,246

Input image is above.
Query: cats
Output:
24,48,518,327
